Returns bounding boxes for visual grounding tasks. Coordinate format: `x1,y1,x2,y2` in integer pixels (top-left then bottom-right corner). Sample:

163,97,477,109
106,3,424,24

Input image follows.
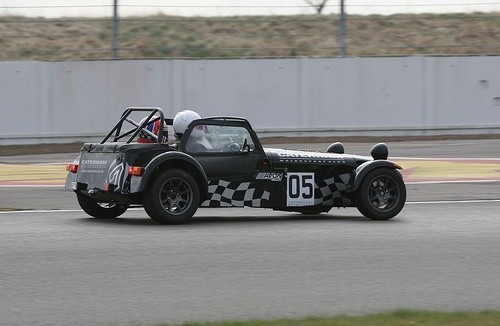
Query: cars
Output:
64,107,408,225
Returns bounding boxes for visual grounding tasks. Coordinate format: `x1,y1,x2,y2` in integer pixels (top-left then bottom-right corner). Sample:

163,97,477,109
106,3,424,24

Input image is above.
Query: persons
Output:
137,115,168,143
172,110,234,152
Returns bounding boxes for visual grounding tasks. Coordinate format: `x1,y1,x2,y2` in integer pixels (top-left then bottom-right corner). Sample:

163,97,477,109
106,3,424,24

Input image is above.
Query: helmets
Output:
138,116,167,140
172,110,205,139
